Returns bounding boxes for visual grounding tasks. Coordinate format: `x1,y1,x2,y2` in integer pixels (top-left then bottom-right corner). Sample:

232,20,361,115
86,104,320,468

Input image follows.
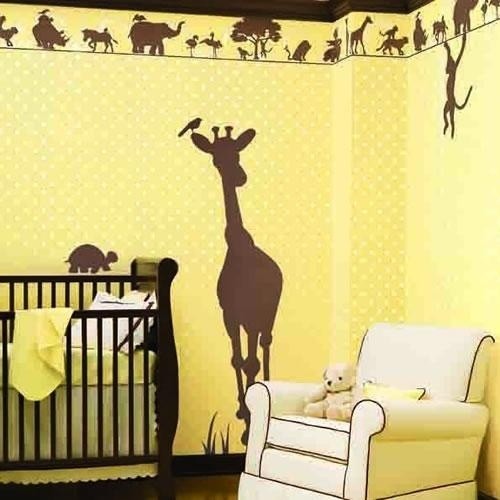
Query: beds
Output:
0,252,184,500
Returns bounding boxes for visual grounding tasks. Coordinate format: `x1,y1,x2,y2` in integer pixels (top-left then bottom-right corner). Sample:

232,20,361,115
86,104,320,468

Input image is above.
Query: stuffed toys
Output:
304,363,356,422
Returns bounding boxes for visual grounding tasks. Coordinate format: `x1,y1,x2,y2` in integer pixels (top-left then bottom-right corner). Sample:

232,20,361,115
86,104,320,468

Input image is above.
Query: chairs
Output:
234,320,498,500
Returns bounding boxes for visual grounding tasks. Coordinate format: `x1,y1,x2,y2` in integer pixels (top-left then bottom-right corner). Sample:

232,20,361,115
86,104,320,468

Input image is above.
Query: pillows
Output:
63,288,156,353
359,376,427,409
119,285,161,357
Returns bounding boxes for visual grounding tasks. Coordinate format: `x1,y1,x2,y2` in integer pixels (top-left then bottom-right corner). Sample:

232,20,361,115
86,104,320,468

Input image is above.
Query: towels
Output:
9,306,76,403
